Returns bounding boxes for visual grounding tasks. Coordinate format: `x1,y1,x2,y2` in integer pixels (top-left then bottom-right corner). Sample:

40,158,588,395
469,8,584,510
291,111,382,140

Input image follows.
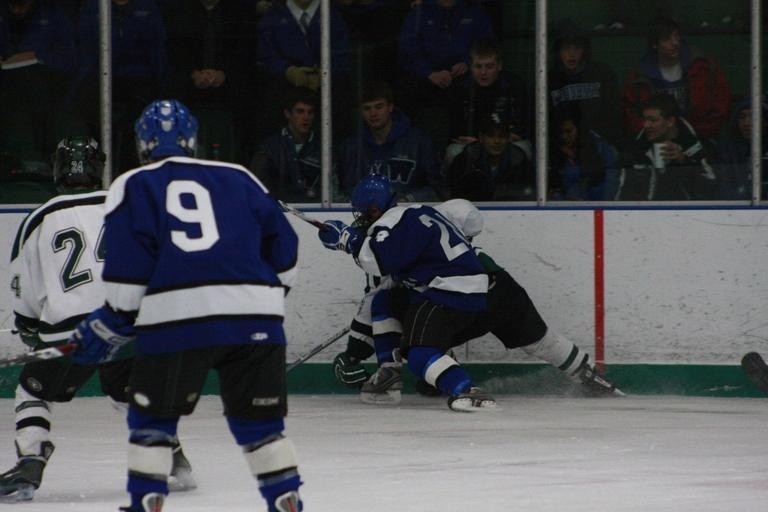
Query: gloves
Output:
67,306,136,365
319,220,363,257
15,320,40,347
334,352,370,388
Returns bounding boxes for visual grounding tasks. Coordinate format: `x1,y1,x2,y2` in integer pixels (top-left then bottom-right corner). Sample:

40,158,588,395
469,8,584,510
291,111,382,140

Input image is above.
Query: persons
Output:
66,94,306,512
2,132,198,504
1,0,767,210
331,193,634,404
316,171,501,416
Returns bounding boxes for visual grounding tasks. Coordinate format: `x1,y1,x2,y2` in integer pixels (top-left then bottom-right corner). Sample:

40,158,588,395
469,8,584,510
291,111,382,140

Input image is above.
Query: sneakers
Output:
448,387,495,412
0,441,54,495
361,369,403,393
170,444,192,476
579,364,615,396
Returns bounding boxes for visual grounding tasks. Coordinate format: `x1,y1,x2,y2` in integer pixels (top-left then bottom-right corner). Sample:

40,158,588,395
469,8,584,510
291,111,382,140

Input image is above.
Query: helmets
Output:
135,99,199,167
351,174,399,235
54,135,105,194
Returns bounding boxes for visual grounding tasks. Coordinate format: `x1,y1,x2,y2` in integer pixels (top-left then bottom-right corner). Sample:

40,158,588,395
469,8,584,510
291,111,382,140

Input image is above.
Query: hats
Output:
480,113,509,130
